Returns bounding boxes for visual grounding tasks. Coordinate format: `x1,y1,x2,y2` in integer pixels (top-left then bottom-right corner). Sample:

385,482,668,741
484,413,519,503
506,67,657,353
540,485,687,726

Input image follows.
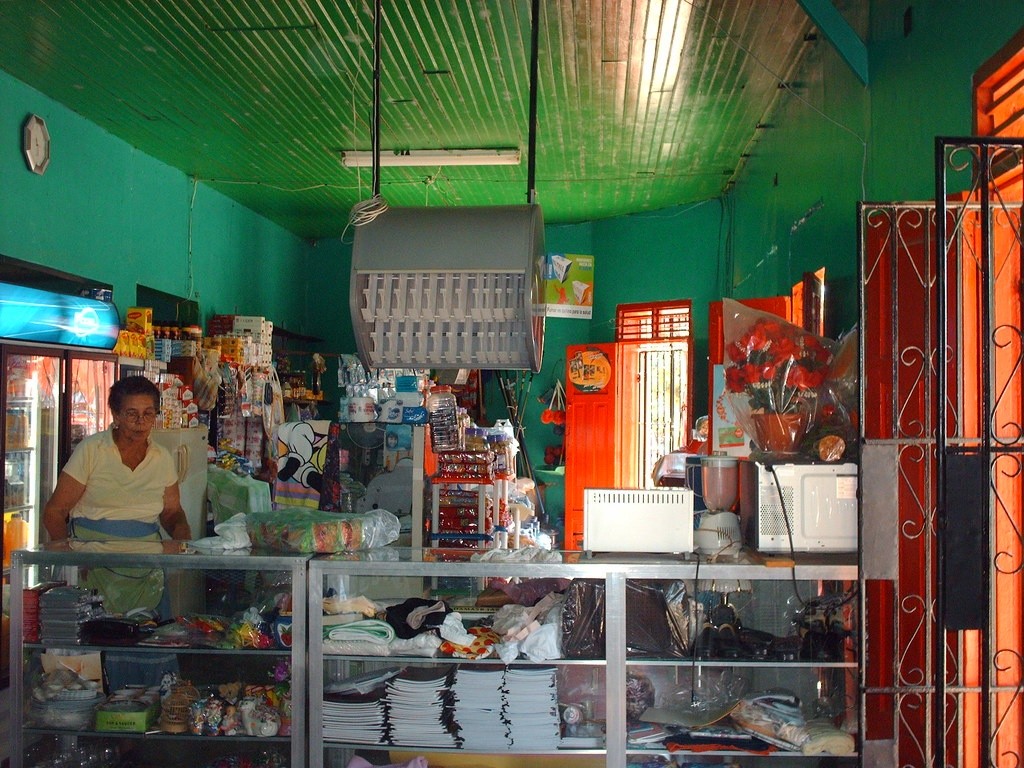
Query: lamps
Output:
341,150,521,168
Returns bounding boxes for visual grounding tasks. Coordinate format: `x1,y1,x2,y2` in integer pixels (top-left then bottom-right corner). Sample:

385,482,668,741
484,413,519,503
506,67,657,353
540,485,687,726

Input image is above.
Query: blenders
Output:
686,450,742,555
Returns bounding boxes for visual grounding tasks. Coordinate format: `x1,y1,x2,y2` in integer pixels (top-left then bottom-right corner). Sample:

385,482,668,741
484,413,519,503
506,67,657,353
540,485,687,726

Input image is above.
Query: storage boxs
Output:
95,684,163,732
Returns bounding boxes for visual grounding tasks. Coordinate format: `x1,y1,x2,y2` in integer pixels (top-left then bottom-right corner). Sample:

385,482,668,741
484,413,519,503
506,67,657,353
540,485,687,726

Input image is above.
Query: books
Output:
321,663,808,752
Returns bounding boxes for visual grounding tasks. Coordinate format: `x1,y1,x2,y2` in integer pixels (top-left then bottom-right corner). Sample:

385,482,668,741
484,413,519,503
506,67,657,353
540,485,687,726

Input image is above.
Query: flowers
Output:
724,318,831,412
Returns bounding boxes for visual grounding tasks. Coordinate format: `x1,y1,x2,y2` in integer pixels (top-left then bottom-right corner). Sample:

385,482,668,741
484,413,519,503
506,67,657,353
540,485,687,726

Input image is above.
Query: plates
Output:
30,691,106,713
126,684,146,689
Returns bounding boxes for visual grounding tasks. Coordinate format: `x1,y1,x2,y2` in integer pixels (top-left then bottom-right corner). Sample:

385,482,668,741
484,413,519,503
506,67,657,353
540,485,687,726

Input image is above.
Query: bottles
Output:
7,514,28,567
3,521,10,568
429,386,470,453
281,370,306,403
5,407,28,449
152,325,204,361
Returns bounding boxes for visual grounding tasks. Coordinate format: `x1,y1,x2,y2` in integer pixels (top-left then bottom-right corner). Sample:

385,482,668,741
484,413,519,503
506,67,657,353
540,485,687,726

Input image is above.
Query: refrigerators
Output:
0,253,121,762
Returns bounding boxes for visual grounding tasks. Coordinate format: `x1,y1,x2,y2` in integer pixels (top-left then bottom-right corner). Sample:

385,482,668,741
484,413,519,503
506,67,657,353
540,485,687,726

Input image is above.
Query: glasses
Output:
118,409,157,423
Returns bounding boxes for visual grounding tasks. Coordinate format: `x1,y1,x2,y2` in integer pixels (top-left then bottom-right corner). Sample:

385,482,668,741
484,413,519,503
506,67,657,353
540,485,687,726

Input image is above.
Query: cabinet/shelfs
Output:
10,535,858,768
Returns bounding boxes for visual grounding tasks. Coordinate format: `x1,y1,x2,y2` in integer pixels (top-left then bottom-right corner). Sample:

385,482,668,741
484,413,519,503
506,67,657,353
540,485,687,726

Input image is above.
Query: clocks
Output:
23,114,50,175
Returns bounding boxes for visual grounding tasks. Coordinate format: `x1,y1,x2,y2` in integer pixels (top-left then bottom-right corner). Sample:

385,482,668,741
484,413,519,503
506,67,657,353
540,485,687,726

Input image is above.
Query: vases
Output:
752,413,808,456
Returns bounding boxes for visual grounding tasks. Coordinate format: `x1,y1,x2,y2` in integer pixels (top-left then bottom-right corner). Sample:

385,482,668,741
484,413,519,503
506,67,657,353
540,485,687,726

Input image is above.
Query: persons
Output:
43,376,191,621
